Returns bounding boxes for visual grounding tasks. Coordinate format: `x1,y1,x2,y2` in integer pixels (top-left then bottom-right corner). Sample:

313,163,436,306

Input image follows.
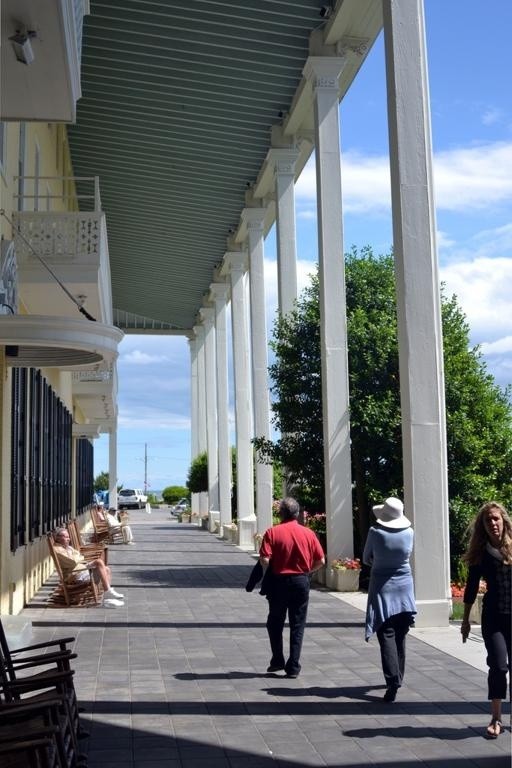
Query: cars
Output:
96,489,109,510
171,498,190,516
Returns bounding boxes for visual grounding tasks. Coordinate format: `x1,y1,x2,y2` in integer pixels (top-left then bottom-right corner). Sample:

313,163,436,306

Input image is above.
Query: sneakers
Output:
383,684,399,703
265,662,299,679
125,540,136,545
102,588,124,606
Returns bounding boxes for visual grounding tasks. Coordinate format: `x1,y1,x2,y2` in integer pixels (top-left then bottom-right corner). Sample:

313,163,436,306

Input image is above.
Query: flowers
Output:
331,557,361,569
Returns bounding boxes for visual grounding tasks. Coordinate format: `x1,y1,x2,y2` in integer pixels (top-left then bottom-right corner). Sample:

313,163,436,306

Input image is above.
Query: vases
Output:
331,569,361,591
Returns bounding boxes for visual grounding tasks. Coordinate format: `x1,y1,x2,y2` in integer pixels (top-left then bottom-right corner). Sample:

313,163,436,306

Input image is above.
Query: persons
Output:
245,496,325,679
460,502,512,738
97,505,105,519
105,506,136,545
53,528,125,608
362,497,418,704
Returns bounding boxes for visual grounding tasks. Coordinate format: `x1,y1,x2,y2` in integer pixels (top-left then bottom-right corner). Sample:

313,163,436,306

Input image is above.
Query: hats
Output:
372,497,413,533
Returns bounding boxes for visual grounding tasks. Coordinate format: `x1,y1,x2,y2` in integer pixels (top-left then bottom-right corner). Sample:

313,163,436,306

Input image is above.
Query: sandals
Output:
485,715,504,737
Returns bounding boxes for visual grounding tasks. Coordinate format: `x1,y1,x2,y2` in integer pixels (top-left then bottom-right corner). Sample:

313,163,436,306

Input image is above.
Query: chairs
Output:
45,518,108,609
1,621,90,768
90,505,114,545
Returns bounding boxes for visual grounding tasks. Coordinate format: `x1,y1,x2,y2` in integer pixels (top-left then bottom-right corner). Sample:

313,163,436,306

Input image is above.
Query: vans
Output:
117,488,147,510
